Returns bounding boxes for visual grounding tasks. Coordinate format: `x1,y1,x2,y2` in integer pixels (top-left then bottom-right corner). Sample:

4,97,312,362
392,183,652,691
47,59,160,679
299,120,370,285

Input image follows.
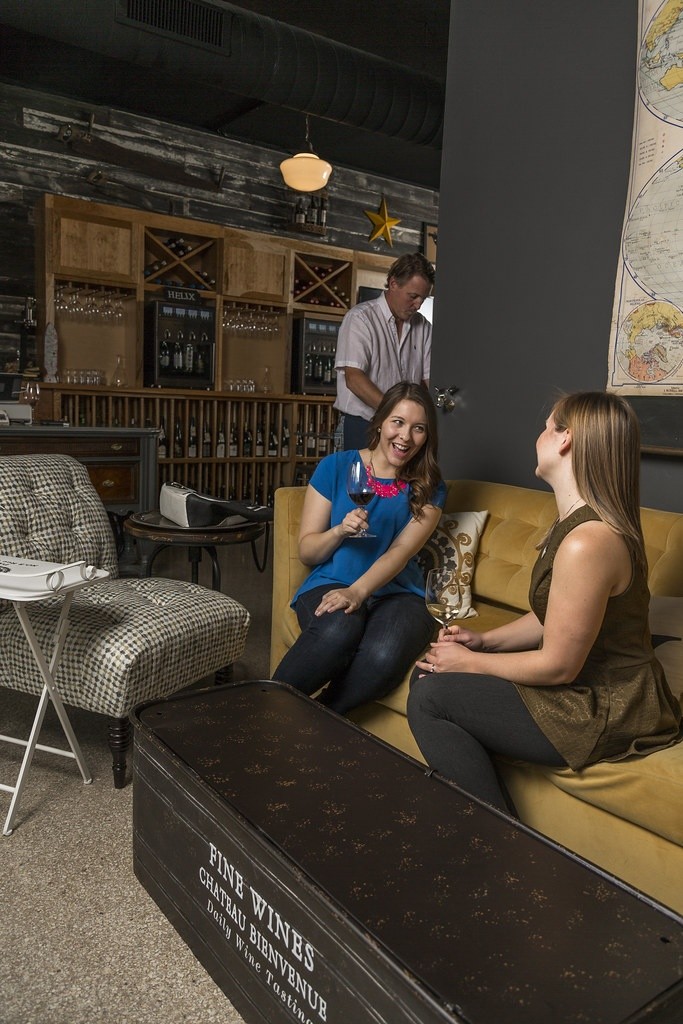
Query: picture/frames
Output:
421,221,438,263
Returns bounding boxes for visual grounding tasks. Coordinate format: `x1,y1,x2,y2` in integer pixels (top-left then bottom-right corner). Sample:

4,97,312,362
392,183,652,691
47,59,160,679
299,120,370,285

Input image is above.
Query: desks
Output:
122,511,268,590
0,571,110,836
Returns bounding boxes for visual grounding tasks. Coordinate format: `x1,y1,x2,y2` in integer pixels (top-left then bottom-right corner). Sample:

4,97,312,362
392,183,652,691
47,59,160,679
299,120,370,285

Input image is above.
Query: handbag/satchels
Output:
158,479,272,574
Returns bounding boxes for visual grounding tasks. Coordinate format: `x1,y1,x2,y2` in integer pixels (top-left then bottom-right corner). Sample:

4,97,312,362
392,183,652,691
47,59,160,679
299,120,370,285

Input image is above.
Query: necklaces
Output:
541,498,582,559
365,465,407,498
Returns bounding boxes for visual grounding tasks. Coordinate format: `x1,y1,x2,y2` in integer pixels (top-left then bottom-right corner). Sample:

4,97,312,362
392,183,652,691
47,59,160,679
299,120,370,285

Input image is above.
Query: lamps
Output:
280,113,333,190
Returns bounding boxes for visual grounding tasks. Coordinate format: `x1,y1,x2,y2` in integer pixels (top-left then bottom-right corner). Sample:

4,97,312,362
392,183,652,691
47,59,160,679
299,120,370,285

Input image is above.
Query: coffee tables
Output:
129,681,683,1024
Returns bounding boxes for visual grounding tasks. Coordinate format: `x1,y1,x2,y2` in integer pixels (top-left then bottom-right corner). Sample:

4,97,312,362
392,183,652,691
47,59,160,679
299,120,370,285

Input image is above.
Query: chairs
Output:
0,453,252,789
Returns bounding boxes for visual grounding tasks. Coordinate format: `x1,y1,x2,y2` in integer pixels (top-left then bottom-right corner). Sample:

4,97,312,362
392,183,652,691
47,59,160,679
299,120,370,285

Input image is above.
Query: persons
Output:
406,392,683,818
332,253,435,453
271,380,448,719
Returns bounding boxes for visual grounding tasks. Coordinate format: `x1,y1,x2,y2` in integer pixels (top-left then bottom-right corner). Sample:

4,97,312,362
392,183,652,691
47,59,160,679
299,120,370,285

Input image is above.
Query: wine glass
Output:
111,355,129,388
22,383,41,426
55,284,127,325
347,461,377,539
261,365,272,395
224,377,256,393
223,307,281,335
425,568,462,636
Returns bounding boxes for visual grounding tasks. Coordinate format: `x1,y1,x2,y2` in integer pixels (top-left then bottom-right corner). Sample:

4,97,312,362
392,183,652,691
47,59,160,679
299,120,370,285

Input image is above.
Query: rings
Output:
431,665,435,671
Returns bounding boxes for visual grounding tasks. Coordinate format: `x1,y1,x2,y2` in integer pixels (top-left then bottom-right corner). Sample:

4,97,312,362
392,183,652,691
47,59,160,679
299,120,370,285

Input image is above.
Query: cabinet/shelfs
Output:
0,424,161,576
39,191,394,400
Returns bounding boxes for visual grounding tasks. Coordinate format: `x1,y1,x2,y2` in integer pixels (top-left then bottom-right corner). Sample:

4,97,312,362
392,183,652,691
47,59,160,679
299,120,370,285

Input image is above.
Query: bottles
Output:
64,413,330,456
145,261,167,276
305,341,337,385
194,270,215,287
295,195,327,226
167,237,192,256
295,267,350,308
63,369,106,386
203,483,273,508
161,328,210,377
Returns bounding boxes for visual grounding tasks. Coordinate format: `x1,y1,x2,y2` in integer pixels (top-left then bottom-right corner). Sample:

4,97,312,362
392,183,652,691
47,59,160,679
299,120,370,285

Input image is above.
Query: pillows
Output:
648,592,683,700
417,510,492,617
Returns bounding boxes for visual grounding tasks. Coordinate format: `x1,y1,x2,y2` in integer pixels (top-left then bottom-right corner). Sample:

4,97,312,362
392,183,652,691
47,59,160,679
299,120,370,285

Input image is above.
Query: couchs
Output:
269,481,682,916
